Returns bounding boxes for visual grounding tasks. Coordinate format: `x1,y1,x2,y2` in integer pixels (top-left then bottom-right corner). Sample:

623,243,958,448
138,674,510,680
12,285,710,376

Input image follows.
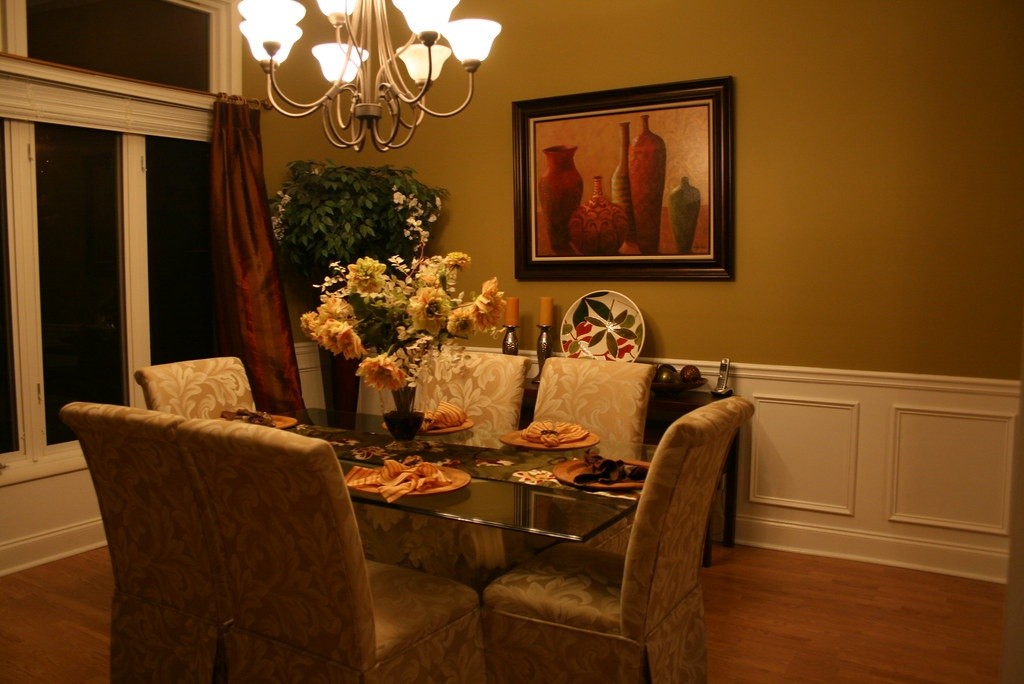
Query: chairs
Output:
57,347,755,684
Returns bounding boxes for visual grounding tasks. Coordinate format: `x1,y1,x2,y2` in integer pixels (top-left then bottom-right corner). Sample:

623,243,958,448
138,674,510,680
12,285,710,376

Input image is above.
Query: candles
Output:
506,295,519,324
539,296,553,325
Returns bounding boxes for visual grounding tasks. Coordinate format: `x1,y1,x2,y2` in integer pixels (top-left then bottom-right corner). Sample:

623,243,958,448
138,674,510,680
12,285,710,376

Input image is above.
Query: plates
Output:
212,413,298,431
552,458,650,489
347,462,472,496
500,424,601,450
560,289,647,363
650,378,709,397
381,419,474,436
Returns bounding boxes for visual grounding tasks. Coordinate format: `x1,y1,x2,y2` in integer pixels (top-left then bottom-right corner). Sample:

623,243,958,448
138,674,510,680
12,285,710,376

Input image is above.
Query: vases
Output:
383,386,426,445
310,300,359,427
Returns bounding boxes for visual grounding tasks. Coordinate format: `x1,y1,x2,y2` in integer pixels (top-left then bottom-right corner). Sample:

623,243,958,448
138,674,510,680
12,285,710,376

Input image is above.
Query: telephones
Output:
711,358,734,399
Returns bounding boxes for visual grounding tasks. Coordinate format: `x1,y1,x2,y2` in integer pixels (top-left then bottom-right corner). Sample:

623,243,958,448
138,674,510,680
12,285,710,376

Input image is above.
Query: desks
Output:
520,378,741,569
253,406,640,587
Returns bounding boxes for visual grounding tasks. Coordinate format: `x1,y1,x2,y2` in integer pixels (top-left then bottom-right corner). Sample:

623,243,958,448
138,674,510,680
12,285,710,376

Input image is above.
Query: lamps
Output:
236,0,503,152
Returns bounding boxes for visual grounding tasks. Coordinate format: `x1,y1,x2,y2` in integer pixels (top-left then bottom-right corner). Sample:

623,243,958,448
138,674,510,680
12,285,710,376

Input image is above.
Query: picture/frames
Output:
507,76,737,283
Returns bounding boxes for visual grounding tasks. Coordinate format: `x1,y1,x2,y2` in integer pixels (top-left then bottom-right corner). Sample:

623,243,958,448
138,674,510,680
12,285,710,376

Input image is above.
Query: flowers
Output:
260,159,449,288
299,187,510,393
